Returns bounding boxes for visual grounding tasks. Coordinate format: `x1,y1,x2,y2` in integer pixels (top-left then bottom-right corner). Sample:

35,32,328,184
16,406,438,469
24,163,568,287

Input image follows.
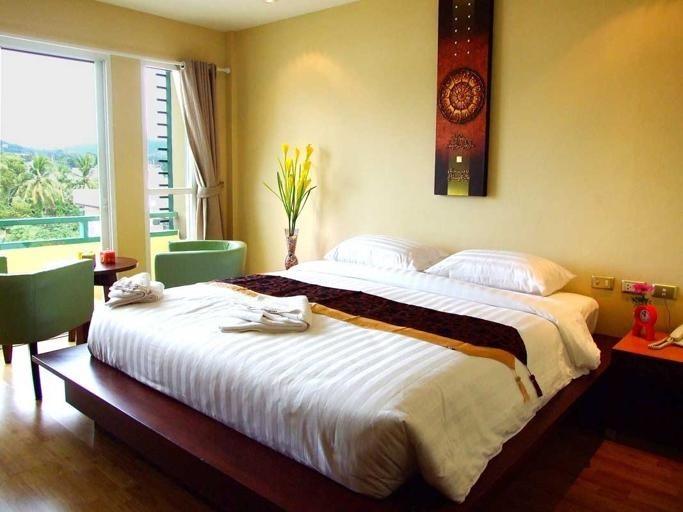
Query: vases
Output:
282,227,298,270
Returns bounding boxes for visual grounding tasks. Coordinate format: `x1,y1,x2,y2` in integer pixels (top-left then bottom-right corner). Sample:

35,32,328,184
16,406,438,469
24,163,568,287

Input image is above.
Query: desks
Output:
67,256,138,345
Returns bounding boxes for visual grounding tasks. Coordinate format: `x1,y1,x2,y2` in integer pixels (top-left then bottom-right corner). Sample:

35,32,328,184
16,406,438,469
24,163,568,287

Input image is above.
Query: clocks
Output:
632,305,658,339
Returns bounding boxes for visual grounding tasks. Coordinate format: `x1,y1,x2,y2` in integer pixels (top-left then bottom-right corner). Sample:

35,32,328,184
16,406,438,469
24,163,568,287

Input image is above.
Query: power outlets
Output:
621,280,644,293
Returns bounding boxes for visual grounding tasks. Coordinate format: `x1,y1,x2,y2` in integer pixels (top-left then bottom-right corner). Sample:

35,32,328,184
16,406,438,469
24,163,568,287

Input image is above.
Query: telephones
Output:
667,324,683,347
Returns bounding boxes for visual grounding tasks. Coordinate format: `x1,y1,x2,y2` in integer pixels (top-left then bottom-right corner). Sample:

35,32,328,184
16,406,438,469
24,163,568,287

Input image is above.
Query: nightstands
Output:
606,327,682,456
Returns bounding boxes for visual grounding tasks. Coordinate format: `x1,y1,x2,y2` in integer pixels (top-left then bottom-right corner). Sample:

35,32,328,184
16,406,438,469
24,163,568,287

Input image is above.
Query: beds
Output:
28,258,619,510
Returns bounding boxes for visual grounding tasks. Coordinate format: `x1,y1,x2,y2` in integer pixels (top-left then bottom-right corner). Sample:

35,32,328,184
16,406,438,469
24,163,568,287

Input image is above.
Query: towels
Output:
216,294,314,333
134,281,164,303
104,272,150,310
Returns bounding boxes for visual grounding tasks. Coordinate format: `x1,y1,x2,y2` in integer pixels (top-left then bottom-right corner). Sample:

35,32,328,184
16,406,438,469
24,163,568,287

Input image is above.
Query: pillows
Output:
322,233,450,274
420,247,577,300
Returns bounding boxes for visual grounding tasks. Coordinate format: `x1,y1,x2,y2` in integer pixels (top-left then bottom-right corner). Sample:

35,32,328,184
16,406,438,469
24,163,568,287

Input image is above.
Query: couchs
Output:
154,240,247,288
0,256,93,364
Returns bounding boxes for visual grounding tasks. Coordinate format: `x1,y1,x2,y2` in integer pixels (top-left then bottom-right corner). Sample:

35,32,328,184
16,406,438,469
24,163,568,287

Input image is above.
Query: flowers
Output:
260,143,317,234
628,281,655,307
